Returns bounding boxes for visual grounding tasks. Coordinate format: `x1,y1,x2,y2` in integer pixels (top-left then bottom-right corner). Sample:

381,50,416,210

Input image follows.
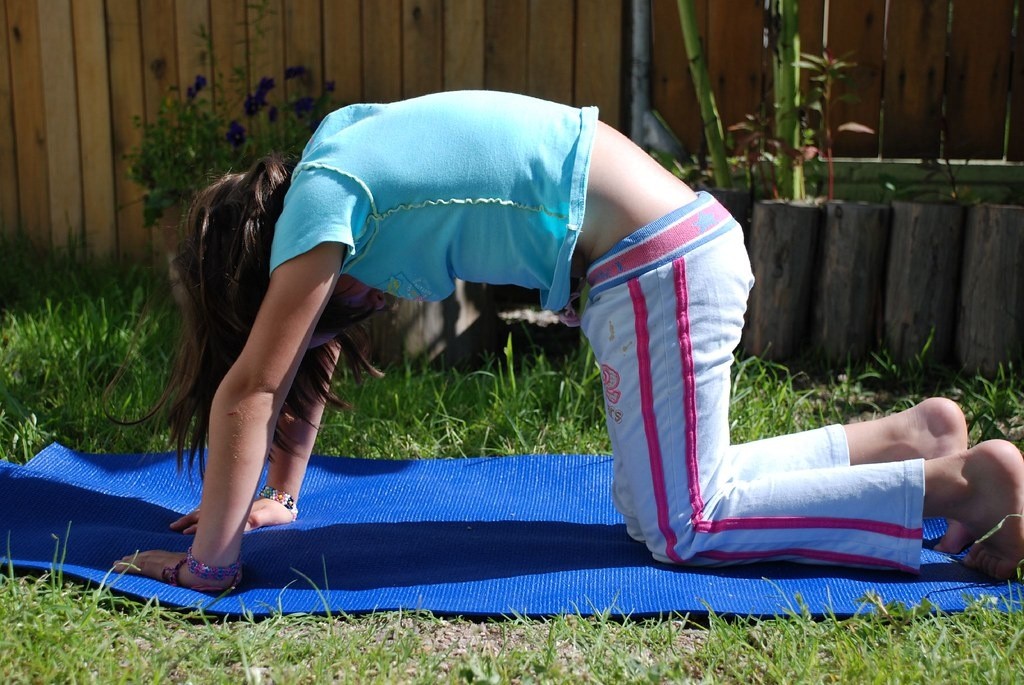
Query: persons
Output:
113,90,1024,590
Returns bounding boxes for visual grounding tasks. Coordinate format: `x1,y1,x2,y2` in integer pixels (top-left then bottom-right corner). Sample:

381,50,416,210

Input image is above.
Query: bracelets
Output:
259,485,298,521
161,546,241,591
